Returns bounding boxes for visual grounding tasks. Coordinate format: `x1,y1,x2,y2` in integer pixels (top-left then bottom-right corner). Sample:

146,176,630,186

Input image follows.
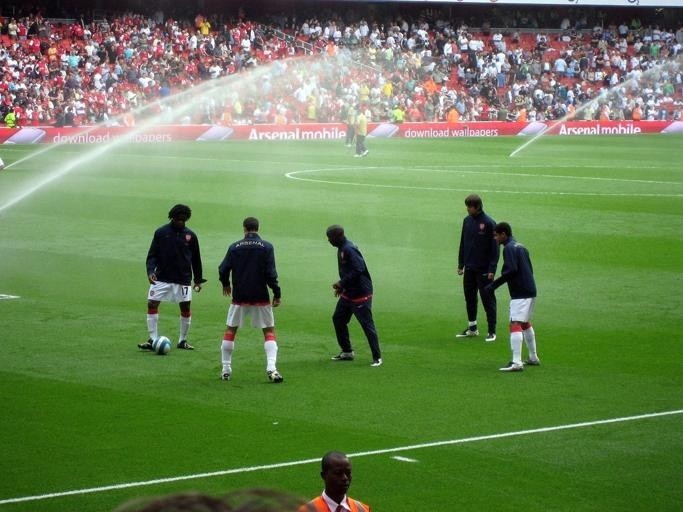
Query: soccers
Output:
153,335,171,355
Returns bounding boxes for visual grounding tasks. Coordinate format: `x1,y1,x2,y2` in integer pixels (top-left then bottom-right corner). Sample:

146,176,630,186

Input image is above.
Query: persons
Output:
484,221,541,372
344,104,357,148
325,225,383,367
217,217,284,382
456,194,500,342
352,109,369,158
0,13,683,129
294,452,372,512
137,205,207,350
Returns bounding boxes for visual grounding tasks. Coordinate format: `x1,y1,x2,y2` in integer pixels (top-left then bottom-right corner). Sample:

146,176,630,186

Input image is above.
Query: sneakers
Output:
523,356,540,365
485,332,496,342
456,328,480,338
331,350,354,361
354,149,369,157
498,361,524,371
369,358,383,367
220,373,231,380
176,340,195,350
266,369,284,382
138,338,153,350
